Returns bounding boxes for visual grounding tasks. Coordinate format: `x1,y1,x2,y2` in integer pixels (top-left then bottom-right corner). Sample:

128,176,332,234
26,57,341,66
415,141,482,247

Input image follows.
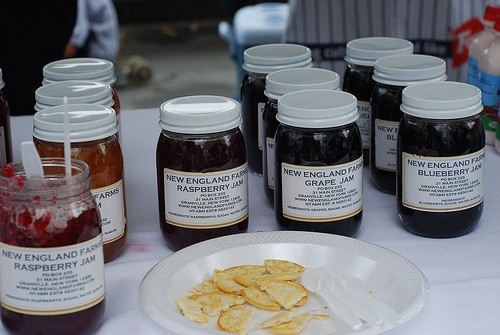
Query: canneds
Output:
0,158,107,335
396,81,486,238
42,57,121,137
275,89,364,237
0,69,13,168
31,103,127,264
262,67,341,207
241,44,312,178
34,79,114,110
156,94,249,252
342,37,414,167
368,54,447,196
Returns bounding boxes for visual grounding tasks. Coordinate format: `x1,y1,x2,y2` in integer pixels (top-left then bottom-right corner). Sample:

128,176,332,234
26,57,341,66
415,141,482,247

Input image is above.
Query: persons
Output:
66,0,120,61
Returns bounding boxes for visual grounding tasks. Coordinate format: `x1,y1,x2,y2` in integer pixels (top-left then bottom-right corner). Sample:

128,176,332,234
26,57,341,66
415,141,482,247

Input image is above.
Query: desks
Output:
0,103,500,335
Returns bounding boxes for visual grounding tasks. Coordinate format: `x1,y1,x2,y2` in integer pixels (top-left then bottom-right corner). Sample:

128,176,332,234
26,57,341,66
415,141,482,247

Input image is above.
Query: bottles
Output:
241,43,320,179
395,81,487,239
263,67,344,206
155,94,249,253
458,5,500,154
0,57,129,335
341,36,413,166
369,54,450,195
273,89,368,237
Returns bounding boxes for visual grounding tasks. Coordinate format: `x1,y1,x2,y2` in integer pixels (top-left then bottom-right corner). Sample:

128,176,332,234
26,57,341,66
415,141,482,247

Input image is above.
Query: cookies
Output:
176,261,332,334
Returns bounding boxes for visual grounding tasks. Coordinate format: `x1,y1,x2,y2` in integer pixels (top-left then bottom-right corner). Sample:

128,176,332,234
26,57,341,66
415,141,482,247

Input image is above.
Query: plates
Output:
139,230,431,335
